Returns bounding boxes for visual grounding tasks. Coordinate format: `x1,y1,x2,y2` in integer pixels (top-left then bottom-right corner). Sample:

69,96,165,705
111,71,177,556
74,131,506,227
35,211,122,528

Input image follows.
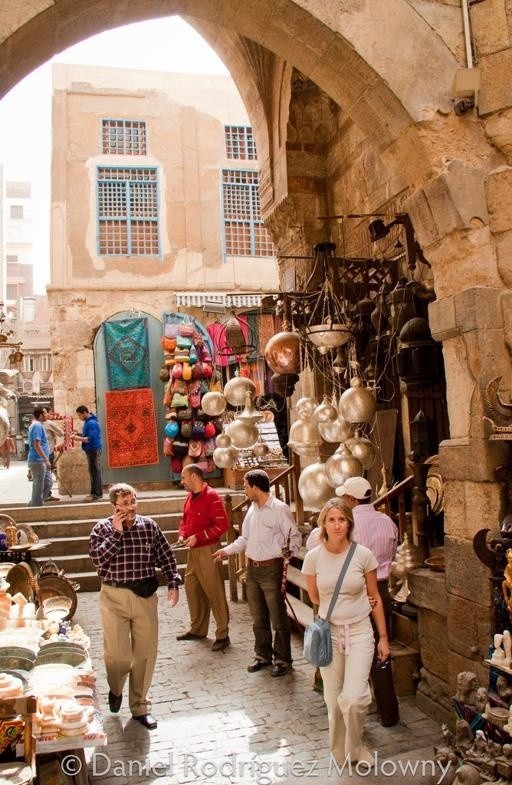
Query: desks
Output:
0,543,52,566
0,624,106,784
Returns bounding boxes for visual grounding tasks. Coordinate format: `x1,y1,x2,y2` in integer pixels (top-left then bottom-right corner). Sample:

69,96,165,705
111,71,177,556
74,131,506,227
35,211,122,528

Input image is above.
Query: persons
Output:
25,406,52,507
176,463,230,652
305,474,400,729
69,405,103,501
89,481,183,730
36,406,65,472
299,496,390,769
210,469,303,677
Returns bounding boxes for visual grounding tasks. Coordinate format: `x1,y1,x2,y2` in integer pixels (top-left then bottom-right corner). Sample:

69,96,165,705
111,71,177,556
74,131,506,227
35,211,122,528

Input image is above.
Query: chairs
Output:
0,696,39,785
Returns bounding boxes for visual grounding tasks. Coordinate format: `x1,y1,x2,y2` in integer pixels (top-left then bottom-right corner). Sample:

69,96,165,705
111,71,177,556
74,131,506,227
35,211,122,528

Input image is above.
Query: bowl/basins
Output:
1,525,96,741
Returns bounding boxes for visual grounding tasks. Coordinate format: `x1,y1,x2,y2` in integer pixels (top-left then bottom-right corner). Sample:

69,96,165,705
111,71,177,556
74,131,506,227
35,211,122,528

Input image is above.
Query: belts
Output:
248,557,284,567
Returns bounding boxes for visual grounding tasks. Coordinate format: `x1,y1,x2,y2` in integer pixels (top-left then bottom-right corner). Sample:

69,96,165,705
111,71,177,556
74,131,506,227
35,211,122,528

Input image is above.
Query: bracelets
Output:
379,635,388,638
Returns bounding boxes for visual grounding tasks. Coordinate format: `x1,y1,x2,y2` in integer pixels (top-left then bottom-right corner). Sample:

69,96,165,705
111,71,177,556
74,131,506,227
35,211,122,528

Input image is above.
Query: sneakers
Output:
85,493,103,499
358,751,377,768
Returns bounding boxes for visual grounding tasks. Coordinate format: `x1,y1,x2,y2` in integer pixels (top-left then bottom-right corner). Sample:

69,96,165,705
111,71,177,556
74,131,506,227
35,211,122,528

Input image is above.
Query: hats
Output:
335,476,373,499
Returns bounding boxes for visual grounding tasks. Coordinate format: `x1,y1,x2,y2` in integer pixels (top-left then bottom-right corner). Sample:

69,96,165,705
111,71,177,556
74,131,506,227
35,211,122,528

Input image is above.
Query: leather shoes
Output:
247,656,273,672
271,660,292,677
43,495,60,501
133,712,157,728
108,689,123,713
211,636,230,651
176,631,207,640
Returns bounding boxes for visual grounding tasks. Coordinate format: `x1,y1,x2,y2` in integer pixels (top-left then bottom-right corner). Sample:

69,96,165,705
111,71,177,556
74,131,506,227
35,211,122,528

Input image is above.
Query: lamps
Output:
306,242,355,353
214,288,282,356
0,341,23,363
368,214,431,271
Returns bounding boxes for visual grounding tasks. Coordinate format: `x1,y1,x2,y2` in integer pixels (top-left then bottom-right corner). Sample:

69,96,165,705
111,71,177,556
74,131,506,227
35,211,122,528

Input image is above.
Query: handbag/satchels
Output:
301,618,334,667
131,576,159,598
160,321,224,475
